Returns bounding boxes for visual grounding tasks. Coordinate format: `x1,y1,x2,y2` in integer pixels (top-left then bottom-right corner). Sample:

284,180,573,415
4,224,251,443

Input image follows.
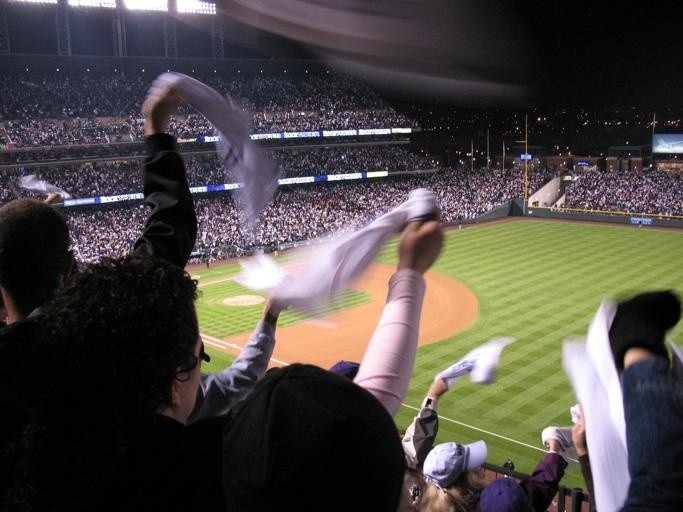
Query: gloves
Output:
608,291,682,365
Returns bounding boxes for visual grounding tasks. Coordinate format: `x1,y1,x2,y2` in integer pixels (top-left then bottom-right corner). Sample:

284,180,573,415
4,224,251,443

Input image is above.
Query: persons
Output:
1,69,683,512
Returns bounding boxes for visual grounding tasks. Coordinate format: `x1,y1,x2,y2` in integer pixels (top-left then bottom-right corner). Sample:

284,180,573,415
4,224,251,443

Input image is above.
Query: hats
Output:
423,439,487,487
480,478,528,512
221,362,405,512
